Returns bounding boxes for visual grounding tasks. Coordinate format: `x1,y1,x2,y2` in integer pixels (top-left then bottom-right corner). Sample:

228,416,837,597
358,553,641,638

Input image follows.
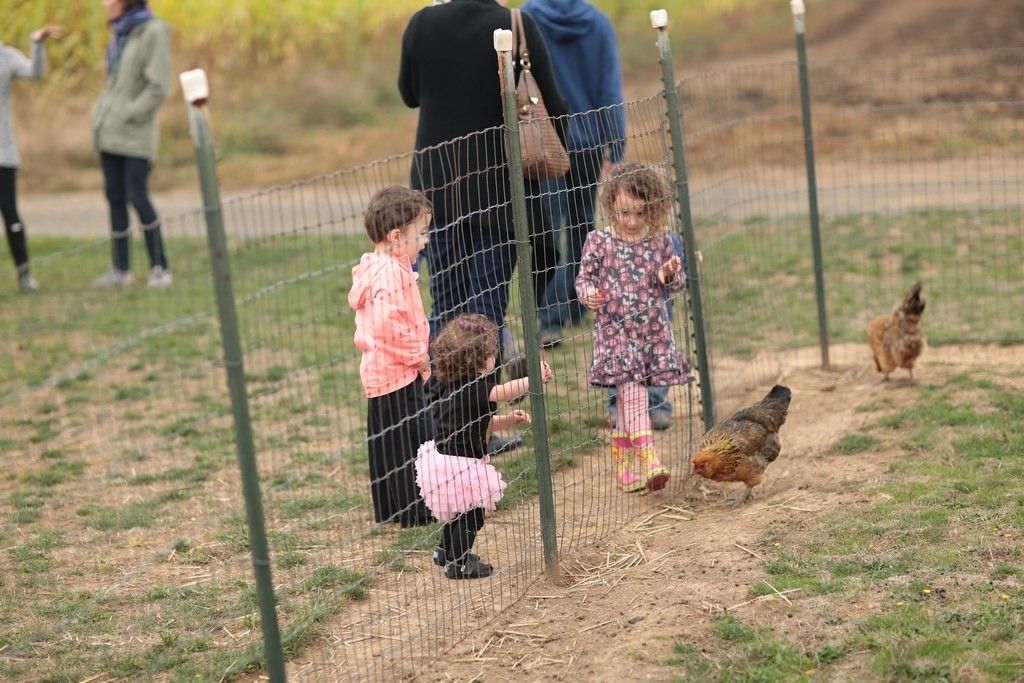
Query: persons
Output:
609,220,705,429
397,0,543,459
89,0,175,289
413,311,555,580
348,183,440,532
517,0,627,348
0,22,65,294
574,161,685,495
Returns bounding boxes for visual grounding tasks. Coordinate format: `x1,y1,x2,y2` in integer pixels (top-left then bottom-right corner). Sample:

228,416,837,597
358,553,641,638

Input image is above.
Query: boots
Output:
609,432,646,490
631,431,669,492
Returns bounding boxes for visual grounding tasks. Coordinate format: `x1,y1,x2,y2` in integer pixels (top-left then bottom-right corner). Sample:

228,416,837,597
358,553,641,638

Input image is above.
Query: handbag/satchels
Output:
502,9,571,181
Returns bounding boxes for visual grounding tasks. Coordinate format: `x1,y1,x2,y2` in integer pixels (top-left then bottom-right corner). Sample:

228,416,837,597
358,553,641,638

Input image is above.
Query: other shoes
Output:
486,430,523,457
90,270,135,287
536,326,561,351
18,276,36,291
147,265,171,289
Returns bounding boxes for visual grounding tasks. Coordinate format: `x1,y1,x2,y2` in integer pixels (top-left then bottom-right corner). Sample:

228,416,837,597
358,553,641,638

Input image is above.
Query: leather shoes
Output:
434,546,481,566
443,556,494,577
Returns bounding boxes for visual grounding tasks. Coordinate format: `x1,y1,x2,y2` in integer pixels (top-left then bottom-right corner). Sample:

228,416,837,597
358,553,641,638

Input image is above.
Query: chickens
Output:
687,384,792,507
867,281,927,384
